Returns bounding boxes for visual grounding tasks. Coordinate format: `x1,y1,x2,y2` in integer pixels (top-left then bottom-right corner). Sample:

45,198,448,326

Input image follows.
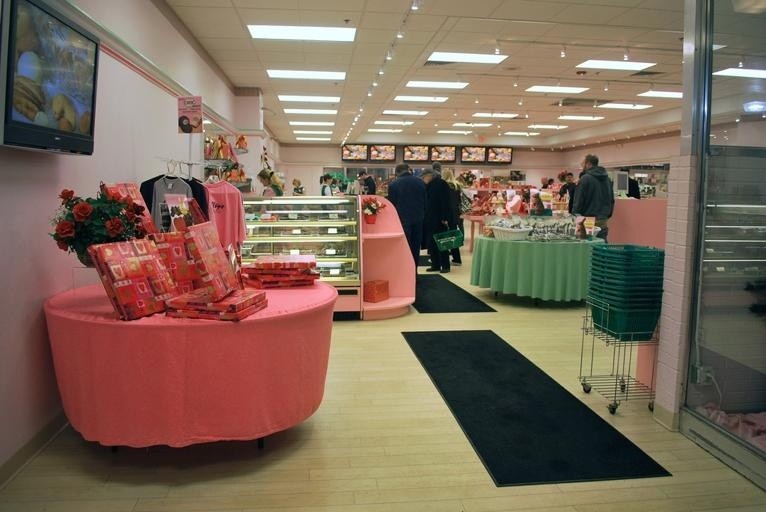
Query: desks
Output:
470,234,606,305
460,214,485,252
41,270,338,454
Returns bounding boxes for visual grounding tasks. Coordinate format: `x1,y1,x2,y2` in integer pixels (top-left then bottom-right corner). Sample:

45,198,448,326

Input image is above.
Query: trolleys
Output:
577,293,660,417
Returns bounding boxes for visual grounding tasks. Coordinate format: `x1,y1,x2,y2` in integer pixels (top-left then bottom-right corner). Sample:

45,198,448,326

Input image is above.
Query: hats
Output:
418,169,434,177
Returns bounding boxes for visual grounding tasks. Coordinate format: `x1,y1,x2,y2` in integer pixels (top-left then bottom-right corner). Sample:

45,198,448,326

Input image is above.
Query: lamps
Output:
338,0,680,150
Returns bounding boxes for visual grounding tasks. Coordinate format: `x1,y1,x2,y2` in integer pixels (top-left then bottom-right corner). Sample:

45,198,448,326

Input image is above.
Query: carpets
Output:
417,255,434,266
400,330,673,486
410,273,497,314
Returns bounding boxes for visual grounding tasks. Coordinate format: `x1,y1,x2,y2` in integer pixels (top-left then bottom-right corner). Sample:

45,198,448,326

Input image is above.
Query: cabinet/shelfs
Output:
701,202,765,281
357,194,415,312
241,198,359,280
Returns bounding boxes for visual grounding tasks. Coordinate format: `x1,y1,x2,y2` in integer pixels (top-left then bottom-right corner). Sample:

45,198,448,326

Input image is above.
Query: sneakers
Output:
426,262,461,273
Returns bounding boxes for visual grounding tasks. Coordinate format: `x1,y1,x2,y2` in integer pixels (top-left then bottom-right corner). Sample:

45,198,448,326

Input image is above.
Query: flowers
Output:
361,197,387,216
46,184,147,252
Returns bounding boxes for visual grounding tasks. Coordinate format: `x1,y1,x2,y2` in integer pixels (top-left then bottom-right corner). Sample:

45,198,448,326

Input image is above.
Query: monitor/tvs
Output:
0,0,101,156
613,171,629,195
342,144,513,163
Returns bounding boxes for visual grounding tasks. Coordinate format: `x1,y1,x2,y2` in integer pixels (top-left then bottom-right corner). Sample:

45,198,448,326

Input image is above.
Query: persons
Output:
620,166,642,199
571,154,614,245
648,172,659,183
292,178,310,218
257,167,284,252
318,173,333,216
331,159,587,274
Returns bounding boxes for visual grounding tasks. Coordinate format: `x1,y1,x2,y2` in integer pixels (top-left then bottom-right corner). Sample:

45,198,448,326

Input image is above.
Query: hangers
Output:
160,158,226,186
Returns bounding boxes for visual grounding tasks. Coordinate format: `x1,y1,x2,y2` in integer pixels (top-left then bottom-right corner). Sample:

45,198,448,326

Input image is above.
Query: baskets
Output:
433,230,463,252
588,245,664,341
490,225,533,241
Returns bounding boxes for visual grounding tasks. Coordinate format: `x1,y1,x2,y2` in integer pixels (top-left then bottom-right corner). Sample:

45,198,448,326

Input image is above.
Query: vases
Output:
73,239,94,268
364,214,377,224
